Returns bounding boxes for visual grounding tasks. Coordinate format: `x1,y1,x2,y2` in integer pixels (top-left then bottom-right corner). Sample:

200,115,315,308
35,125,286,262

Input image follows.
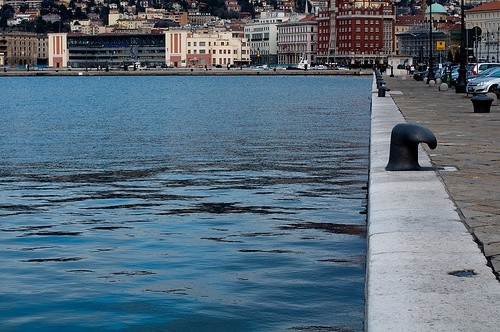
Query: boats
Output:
296,57,346,70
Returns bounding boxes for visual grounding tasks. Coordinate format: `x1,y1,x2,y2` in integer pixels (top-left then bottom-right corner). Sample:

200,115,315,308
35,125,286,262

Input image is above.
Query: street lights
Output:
449,45,461,60
448,5,466,93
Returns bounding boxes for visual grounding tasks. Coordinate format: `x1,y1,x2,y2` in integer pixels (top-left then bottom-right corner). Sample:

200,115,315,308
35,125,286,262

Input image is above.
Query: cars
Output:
466,67,500,82
413,66,460,83
465,67,500,98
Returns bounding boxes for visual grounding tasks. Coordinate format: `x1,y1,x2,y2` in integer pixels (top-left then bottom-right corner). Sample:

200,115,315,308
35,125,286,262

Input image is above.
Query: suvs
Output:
466,62,500,76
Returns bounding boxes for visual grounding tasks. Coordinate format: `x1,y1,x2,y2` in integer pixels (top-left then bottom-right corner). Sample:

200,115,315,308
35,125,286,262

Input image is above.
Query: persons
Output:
205,63,207,71
305,63,308,71
408,65,415,75
227,63,230,70
